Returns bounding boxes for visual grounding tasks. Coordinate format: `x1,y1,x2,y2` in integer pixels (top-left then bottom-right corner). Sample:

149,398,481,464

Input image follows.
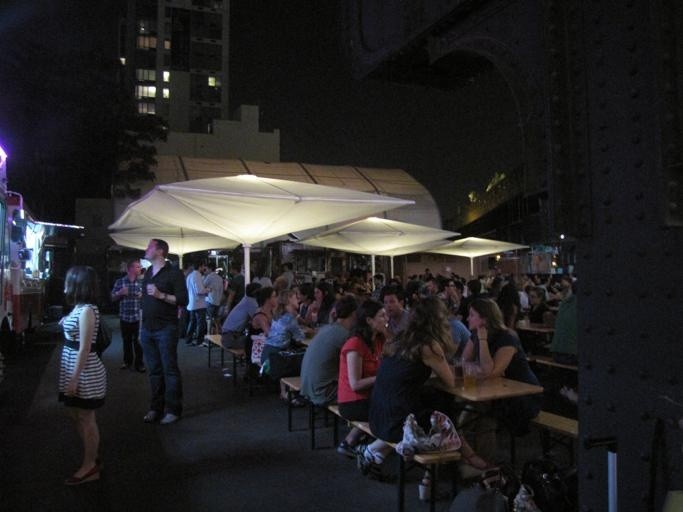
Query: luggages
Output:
521,453,581,512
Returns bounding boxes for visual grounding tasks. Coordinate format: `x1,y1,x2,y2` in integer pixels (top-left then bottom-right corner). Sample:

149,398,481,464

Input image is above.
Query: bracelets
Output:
163,294,167,300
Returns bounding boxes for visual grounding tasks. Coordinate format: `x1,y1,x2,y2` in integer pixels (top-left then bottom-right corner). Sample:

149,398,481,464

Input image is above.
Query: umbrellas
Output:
297,212,460,290
108,230,242,272
111,172,413,284
378,240,454,280
431,237,529,276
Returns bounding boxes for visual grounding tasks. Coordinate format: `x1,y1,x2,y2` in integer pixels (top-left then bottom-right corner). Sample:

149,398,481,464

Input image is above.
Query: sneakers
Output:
134,365,146,373
337,439,386,465
559,385,579,402
119,362,131,370
143,409,181,425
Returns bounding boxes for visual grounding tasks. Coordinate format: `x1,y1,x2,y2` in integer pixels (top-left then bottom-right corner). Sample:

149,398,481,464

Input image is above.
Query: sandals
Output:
280,390,305,408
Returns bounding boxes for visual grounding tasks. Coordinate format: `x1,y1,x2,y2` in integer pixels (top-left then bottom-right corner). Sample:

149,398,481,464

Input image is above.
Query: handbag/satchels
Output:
94,318,113,358
311,379,339,408
268,347,307,377
513,483,542,511
399,411,463,456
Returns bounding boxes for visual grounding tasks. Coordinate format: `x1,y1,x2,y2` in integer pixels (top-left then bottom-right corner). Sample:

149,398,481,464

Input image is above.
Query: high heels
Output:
64,463,101,485
417,467,450,502
454,452,501,480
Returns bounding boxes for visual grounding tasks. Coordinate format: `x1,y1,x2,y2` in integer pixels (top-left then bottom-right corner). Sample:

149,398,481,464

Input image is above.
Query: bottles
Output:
542,311,554,329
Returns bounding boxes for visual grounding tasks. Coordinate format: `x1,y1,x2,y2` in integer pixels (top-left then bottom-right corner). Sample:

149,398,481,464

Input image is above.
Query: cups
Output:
518,316,529,328
453,358,477,389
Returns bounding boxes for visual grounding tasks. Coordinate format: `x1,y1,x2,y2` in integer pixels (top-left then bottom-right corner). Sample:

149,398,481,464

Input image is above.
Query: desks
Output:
298,337,544,467
518,323,557,334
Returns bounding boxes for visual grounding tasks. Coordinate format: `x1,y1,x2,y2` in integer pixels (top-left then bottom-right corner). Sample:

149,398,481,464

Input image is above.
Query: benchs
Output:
539,411,580,460
534,355,580,373
206,335,462,509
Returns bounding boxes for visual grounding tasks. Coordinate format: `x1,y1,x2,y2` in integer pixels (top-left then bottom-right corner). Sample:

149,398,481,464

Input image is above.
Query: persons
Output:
111,260,146,372
138,239,189,424
59,265,106,486
137,267,146,279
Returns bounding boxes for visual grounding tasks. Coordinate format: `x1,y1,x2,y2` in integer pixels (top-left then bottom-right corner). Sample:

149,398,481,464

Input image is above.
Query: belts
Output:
222,331,242,335
